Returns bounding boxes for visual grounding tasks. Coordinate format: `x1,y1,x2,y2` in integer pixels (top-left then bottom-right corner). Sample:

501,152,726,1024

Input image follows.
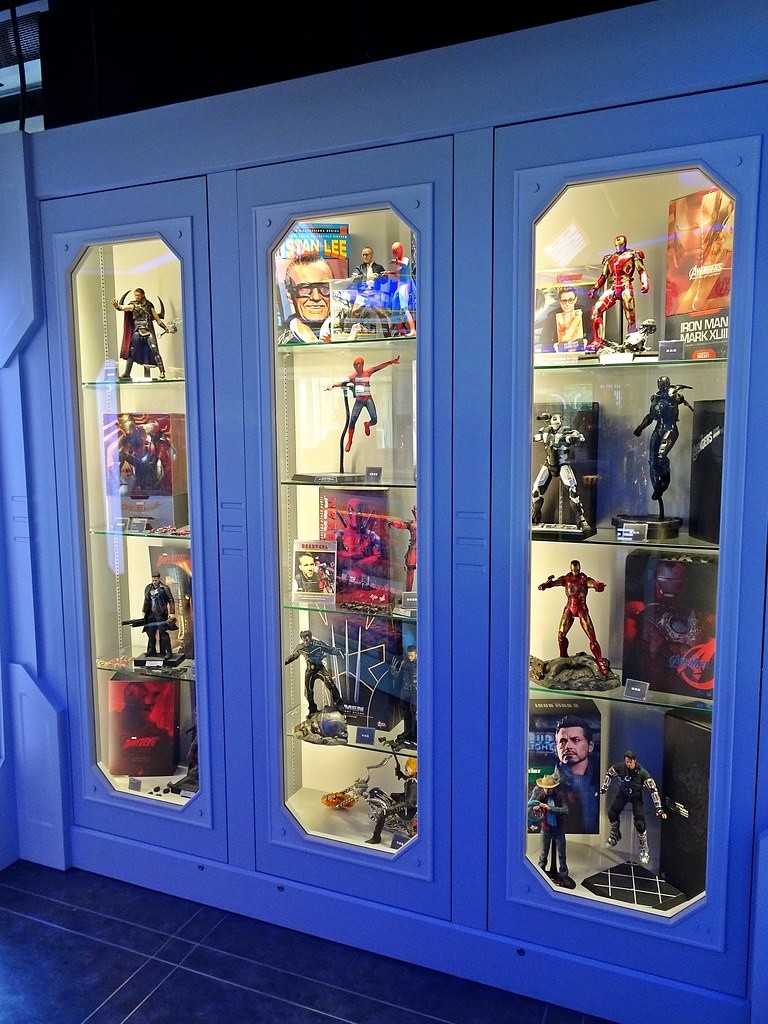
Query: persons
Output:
109,289,170,379
283,630,341,719
282,255,337,343
343,248,398,342
531,414,592,532
141,572,178,660
391,645,418,743
327,356,400,452
524,716,600,834
538,287,594,351
537,560,610,677
585,235,650,351
634,376,684,500
530,774,572,886
294,554,325,593
365,757,417,843
600,751,667,864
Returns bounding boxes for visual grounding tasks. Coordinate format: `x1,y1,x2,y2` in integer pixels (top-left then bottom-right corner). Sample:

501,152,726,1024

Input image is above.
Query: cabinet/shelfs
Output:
0,0,768,1024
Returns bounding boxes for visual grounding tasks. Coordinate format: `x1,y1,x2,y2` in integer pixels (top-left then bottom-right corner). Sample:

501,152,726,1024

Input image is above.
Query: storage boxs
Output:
529,697,601,835
107,496,188,532
103,412,187,496
108,671,181,778
658,703,713,901
622,549,719,701
308,603,406,733
318,487,391,604
